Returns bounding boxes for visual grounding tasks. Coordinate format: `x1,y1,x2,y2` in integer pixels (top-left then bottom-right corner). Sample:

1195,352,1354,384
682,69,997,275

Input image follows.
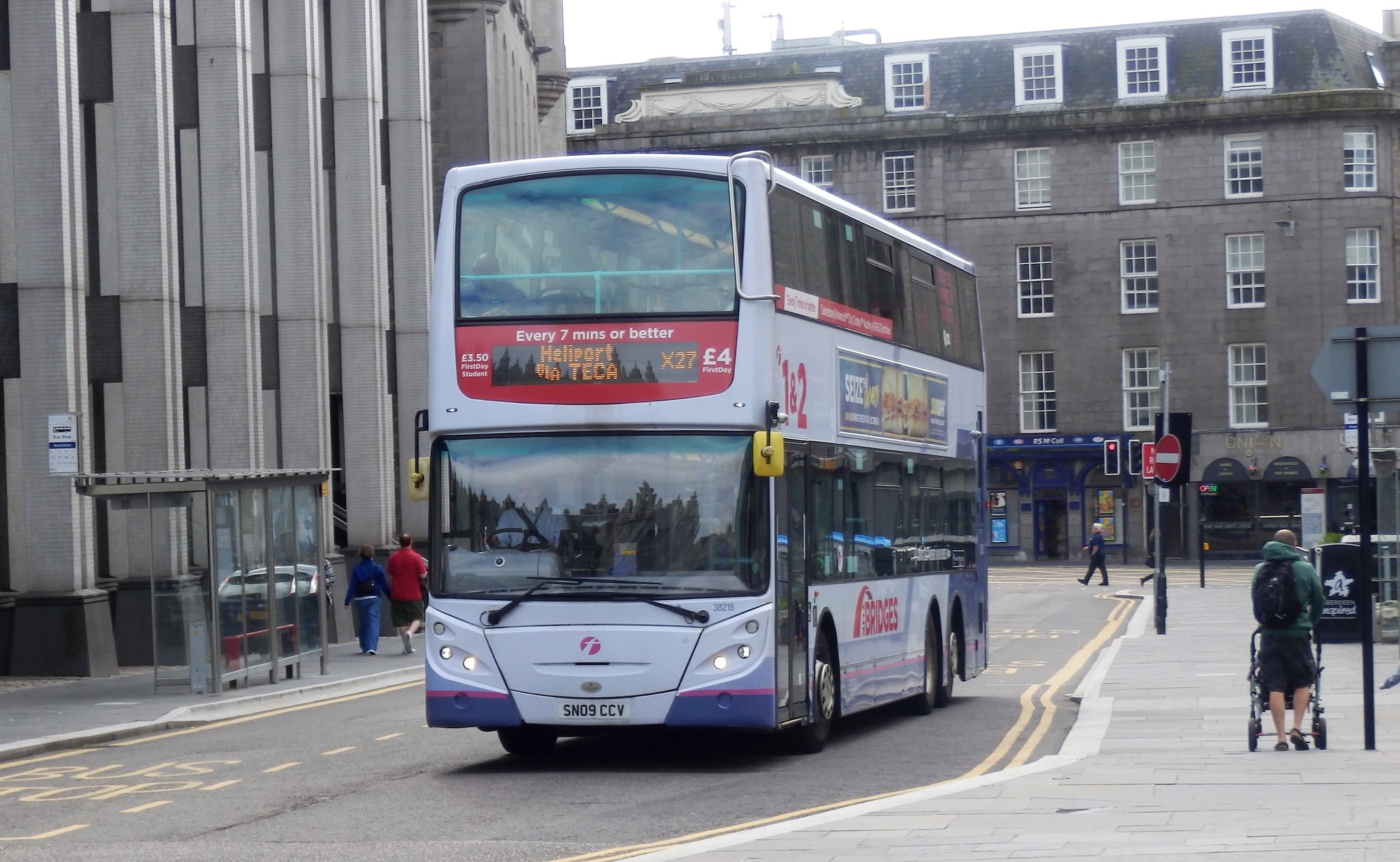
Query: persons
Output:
1140,525,1167,589
1252,529,1326,750
296,545,335,648
460,254,527,320
1078,523,1109,585
493,473,569,554
345,545,391,654
386,534,427,653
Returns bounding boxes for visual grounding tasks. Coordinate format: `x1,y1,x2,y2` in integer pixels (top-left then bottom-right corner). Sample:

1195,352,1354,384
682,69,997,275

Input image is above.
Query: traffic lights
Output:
1102,439,1122,476
1127,439,1142,476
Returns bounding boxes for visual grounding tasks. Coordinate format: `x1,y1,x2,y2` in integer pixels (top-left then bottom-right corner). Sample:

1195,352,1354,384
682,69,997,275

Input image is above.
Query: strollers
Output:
1245,625,1327,753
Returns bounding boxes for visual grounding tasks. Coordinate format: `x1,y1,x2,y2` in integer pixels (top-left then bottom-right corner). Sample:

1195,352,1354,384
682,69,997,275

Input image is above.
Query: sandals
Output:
1289,728,1310,751
1274,741,1288,751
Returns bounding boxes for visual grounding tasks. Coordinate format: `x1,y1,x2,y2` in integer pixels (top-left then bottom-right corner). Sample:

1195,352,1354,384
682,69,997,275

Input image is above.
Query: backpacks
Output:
1252,559,1308,629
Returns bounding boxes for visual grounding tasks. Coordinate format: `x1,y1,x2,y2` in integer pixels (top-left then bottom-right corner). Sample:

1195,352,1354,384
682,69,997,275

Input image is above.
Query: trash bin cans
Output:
1341,533,1400,602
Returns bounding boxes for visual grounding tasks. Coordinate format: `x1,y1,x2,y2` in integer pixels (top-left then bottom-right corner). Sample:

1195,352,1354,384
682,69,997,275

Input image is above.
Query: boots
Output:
1140,574,1154,587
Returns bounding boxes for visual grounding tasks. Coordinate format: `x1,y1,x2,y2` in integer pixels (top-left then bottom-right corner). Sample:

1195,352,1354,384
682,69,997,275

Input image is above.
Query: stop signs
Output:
1152,434,1182,483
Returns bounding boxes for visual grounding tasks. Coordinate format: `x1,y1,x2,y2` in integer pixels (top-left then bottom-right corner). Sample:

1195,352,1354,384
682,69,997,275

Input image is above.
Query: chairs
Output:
525,279,733,313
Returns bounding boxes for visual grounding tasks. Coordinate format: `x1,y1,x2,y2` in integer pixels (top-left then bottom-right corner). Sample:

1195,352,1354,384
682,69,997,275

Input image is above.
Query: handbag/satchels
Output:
359,576,376,594
1145,555,1154,568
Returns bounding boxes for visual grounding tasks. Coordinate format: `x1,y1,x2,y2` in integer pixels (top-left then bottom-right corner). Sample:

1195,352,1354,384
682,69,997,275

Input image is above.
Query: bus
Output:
404,142,992,747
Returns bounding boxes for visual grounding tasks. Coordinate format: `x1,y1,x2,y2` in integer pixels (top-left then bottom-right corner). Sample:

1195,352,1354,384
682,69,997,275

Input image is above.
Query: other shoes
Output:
402,649,416,654
367,650,378,655
1098,582,1108,585
1077,579,1088,585
404,631,412,653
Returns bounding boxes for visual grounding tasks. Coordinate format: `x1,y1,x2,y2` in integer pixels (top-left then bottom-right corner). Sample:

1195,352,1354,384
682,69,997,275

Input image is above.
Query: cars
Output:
220,564,321,628
